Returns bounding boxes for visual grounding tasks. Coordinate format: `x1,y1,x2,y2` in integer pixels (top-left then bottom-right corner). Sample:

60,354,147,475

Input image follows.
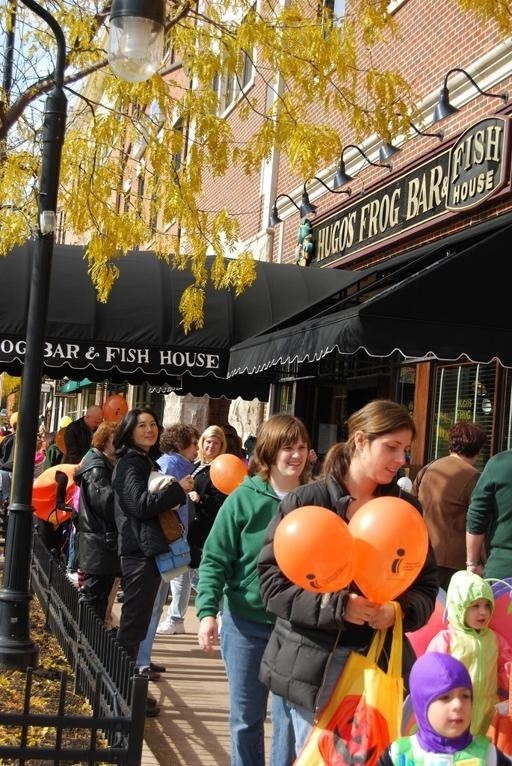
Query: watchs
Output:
467,560,482,566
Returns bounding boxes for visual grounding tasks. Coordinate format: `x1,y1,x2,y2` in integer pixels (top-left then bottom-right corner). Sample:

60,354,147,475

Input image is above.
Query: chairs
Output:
156,620,185,635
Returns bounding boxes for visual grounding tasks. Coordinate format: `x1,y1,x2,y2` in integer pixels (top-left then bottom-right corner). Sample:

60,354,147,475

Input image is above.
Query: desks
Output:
1,0,165,667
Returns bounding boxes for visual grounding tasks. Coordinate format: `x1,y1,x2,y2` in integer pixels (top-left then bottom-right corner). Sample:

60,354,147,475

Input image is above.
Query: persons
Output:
420,570,510,736
258,400,440,765
155,425,227,634
135,423,199,682
110,408,194,717
411,423,485,601
465,451,511,586
195,415,311,763
380,651,510,763
0,405,122,626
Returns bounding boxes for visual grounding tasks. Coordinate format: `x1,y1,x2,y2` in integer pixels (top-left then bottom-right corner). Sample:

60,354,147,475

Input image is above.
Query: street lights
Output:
154,536,191,572
159,510,185,543
292,651,404,765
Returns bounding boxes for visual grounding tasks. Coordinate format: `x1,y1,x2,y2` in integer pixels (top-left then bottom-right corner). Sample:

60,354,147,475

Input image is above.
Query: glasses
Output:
334,145,391,189
435,70,507,121
271,194,299,225
380,113,444,163
300,177,351,218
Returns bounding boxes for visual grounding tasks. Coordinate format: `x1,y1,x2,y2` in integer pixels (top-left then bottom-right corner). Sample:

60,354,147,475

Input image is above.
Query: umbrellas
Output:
142,668,160,680
150,662,165,672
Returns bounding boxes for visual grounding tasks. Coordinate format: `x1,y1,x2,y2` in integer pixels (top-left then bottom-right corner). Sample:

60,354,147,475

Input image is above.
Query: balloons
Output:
348,496,429,603
104,394,128,422
273,506,356,593
10,412,18,428
60,416,72,428
210,454,247,495
